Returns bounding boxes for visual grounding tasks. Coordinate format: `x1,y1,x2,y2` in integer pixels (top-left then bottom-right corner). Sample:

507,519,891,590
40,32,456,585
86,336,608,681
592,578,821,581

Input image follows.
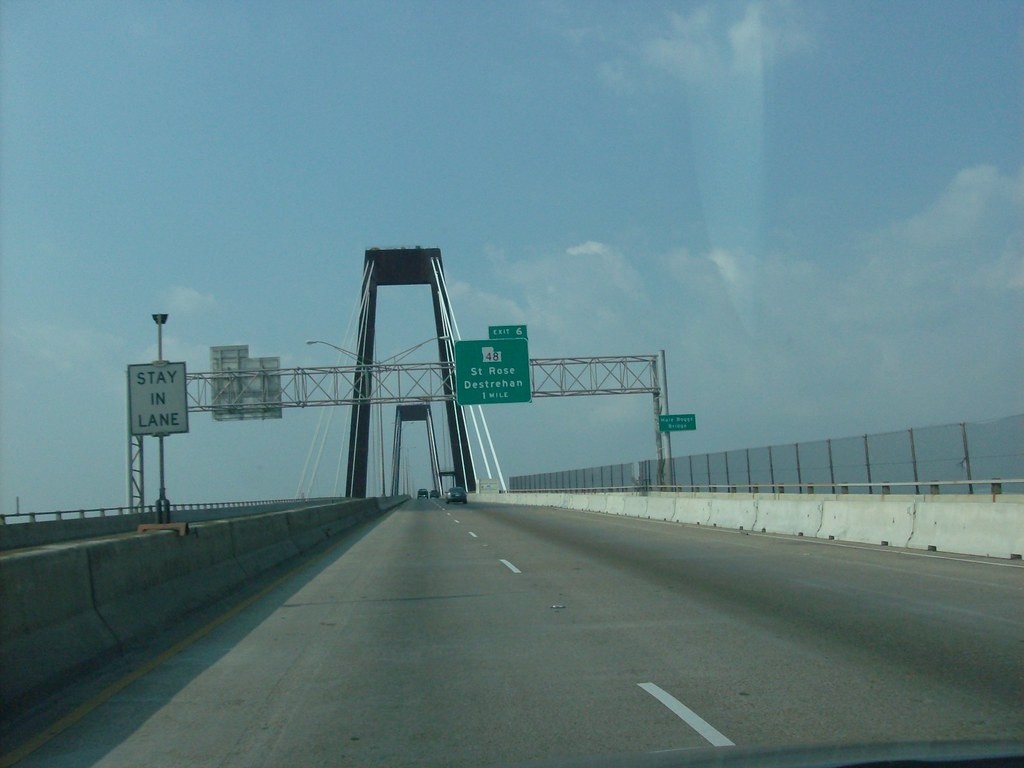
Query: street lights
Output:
305,335,453,498
152,312,171,524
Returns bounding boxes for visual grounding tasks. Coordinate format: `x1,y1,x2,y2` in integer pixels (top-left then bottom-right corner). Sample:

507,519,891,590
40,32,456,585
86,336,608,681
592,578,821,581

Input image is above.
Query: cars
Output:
429,490,440,499
417,488,429,499
445,487,468,505
